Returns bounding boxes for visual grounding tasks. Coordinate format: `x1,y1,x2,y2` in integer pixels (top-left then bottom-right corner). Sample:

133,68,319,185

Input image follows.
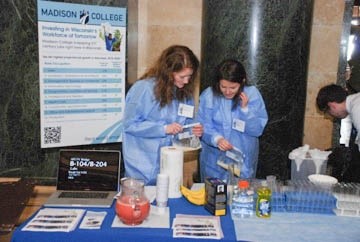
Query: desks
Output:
0,179,359,242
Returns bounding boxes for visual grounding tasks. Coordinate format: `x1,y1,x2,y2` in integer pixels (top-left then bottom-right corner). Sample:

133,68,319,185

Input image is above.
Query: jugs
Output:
115,176,150,226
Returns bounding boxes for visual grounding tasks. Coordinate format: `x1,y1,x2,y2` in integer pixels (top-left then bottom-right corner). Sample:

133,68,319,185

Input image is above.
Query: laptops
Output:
45,149,120,207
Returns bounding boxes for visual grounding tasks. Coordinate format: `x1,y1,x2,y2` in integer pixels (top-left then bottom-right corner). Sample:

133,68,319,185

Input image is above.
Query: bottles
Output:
231,181,254,220
256,180,272,218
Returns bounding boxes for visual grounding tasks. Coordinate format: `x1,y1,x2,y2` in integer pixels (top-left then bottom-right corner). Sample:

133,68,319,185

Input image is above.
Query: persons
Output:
315,83,360,150
121,45,204,186
196,59,270,182
103,25,118,53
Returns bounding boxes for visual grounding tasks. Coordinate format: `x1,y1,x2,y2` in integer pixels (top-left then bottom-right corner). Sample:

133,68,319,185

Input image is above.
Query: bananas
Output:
180,185,205,206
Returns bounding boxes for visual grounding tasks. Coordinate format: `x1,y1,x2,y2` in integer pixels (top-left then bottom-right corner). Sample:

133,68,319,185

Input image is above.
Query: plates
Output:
308,174,338,186
